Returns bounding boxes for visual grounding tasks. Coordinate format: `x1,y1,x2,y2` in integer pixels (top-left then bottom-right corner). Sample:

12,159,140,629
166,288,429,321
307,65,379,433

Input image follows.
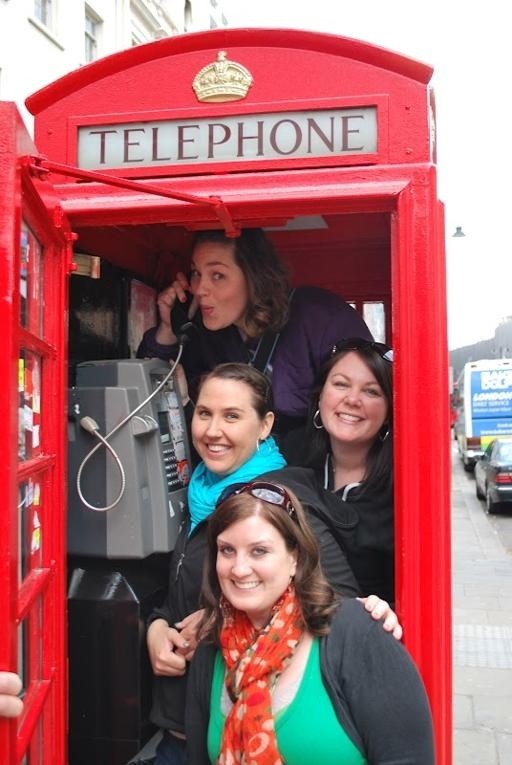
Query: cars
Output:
470,439,512,517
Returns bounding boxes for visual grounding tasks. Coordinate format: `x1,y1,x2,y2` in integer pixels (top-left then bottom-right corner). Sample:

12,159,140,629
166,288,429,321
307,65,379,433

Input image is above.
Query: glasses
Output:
216,483,296,520
330,337,393,362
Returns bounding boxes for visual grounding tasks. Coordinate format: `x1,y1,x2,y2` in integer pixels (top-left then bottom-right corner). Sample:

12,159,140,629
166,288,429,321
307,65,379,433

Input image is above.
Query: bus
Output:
450,357,512,474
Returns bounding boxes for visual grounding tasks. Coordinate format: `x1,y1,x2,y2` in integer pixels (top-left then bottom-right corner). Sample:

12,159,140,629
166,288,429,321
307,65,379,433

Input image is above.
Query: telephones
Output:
167,271,201,346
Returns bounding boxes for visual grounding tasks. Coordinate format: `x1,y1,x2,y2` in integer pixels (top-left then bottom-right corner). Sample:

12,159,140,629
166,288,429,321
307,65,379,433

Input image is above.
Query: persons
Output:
272,336,396,619
125,357,412,765
131,220,378,469
180,477,437,765
0,670,24,720
